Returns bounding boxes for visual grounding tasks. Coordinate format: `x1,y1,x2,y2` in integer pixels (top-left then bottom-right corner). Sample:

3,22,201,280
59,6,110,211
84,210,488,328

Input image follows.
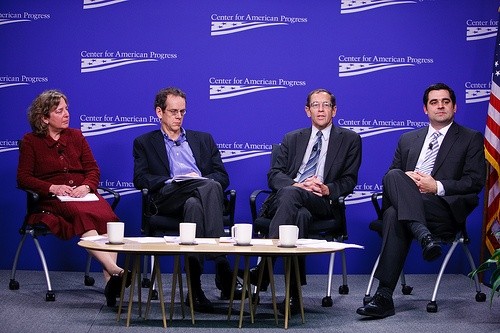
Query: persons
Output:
357,83,486,317
133,87,248,311
237,89,363,316
16,91,132,307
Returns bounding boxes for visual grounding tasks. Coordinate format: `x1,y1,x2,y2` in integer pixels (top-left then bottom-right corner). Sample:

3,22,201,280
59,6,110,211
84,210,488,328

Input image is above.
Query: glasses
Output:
309,101,333,110
168,108,187,116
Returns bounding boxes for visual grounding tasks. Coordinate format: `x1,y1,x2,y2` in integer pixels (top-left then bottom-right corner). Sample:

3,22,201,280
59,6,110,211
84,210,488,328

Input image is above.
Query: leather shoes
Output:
356,293,395,318
237,266,270,291
420,233,441,263
279,296,301,316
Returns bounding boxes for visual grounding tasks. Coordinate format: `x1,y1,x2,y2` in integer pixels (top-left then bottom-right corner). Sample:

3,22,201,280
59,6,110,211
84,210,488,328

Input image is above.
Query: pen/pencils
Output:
52,186,76,197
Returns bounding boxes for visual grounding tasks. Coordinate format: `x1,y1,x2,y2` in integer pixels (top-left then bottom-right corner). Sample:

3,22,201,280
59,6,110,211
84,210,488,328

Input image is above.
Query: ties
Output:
418,131,441,176
298,131,323,184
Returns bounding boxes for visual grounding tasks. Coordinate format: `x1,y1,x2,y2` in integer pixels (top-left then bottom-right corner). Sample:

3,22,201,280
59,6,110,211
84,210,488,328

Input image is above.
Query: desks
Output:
77,236,344,329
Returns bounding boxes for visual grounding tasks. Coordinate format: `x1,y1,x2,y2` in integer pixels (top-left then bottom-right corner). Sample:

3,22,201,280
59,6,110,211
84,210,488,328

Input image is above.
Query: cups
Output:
279,225,299,246
179,223,196,244
232,223,252,245
107,222,124,244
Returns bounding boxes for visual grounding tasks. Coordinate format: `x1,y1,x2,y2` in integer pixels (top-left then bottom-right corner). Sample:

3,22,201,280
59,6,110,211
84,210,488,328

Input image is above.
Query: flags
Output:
484,11,500,265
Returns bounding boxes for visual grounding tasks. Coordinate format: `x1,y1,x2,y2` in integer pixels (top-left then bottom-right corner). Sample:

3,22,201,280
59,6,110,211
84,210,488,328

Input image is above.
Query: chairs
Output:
140,186,236,300
363,191,486,313
9,185,120,302
250,190,352,306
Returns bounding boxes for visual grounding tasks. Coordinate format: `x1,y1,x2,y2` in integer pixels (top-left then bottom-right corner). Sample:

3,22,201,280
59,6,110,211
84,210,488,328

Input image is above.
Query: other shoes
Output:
116,269,132,298
185,291,213,313
104,274,120,307
221,288,245,300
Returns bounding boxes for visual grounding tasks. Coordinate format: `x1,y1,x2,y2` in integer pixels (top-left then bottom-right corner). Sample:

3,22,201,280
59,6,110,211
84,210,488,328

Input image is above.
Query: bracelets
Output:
85,185,90,191
